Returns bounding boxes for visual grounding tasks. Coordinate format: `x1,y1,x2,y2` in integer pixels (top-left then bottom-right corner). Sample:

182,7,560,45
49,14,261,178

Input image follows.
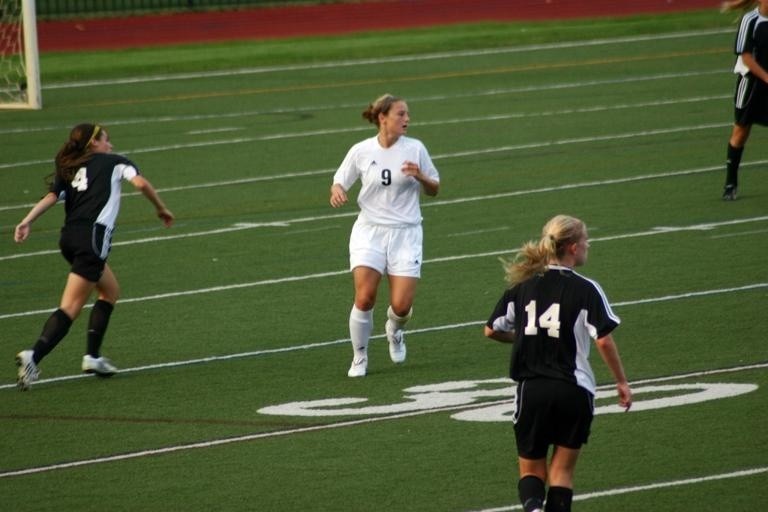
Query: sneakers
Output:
82,353,118,376
384,319,407,364
722,183,739,202
347,355,368,377
15,348,42,390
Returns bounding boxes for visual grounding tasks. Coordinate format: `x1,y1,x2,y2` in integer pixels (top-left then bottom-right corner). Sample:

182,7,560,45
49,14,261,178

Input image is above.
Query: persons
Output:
330,93,440,378
484,215,631,512
720,1,768,200
14,123,175,391
